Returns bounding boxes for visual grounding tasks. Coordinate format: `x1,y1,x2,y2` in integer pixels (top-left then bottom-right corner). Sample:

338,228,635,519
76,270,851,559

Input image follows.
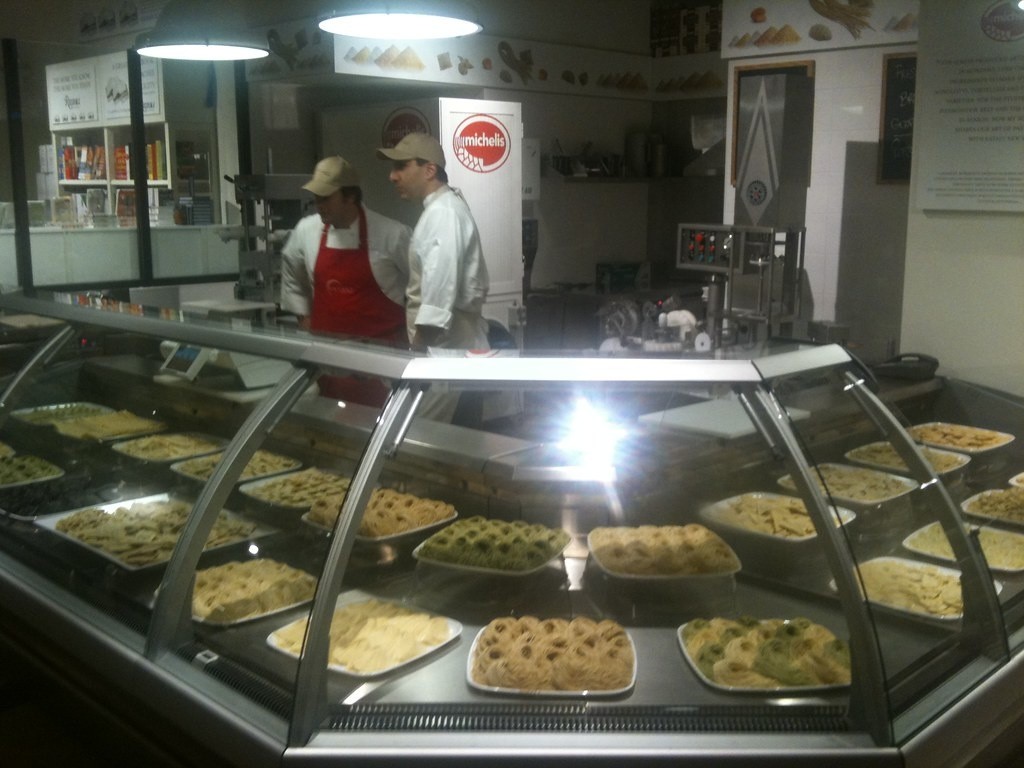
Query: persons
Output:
278,156,414,409
377,134,489,429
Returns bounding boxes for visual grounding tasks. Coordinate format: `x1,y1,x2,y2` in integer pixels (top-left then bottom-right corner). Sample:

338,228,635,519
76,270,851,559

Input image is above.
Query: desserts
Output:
0,423,1024,690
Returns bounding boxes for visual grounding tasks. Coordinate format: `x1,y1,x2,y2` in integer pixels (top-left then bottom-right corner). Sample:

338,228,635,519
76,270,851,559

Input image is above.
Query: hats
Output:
302,155,360,197
375,131,446,169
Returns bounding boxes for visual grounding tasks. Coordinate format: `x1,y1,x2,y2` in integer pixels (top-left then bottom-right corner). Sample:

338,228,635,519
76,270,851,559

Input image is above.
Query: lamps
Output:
319,1,484,42
136,1,273,62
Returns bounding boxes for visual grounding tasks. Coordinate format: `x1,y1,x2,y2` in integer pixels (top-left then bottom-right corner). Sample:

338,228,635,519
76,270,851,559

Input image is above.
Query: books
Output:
58,141,180,322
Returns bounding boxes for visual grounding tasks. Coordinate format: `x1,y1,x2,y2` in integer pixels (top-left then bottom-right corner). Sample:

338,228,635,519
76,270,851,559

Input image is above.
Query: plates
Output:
587,524,741,580
466,624,638,695
829,556,1003,621
412,519,574,577
238,471,351,509
843,439,971,475
154,579,315,627
960,489,1024,525
699,492,856,543
906,420,1015,452
677,622,852,691
266,594,463,678
1008,472,1024,487
902,520,1024,573
778,463,919,506
301,510,458,541
